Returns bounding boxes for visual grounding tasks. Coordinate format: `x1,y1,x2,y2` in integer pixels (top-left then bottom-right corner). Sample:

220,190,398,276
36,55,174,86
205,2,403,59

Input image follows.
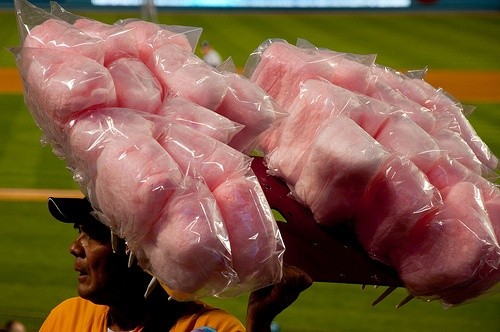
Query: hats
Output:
48,195,108,229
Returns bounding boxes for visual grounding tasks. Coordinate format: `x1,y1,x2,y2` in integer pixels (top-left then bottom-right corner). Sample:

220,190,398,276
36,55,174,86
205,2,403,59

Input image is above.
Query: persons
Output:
199,40,223,72
0,197,314,332
